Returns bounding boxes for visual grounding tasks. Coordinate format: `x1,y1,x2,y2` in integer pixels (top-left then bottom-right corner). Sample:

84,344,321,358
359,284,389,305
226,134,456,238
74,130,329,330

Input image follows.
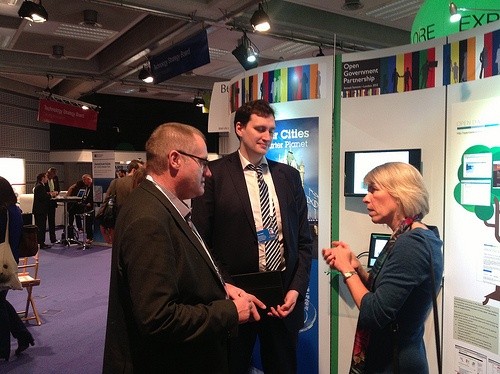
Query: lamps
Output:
232,35,257,71
250,2,271,32
449,2,461,23
193,90,205,107
18,0,48,23
138,65,154,84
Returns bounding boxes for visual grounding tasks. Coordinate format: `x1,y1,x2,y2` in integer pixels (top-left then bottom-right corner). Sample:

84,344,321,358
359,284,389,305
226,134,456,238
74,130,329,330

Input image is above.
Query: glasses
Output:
168,149,210,168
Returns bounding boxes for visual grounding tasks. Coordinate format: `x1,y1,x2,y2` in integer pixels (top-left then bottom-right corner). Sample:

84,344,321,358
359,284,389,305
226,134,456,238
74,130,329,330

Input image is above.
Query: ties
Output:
246,164,282,272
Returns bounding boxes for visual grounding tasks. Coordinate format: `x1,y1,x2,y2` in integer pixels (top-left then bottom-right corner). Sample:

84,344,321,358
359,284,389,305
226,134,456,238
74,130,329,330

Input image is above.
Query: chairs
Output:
16,244,42,326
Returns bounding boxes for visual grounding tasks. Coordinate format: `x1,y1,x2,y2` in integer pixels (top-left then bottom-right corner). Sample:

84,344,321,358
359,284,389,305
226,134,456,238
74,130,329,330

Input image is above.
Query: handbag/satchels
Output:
97,179,118,230
0,208,23,290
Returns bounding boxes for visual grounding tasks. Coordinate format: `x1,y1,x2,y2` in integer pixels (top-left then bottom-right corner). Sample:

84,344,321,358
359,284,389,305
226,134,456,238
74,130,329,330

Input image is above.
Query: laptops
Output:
70,189,85,199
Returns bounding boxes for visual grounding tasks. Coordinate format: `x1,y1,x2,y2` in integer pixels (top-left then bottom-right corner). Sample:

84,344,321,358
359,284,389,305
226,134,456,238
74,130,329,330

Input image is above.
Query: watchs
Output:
342,270,356,284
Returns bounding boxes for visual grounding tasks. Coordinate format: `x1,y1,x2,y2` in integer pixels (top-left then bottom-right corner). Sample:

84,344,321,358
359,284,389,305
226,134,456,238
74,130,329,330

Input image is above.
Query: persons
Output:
101,122,266,374
320,162,444,374
0,176,36,362
64,174,101,244
97,160,145,229
31,172,57,249
191,99,313,374
44,167,61,244
116,167,126,177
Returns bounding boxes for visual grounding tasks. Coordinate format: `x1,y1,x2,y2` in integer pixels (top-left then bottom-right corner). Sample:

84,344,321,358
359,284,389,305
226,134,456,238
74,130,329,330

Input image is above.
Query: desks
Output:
51,196,84,247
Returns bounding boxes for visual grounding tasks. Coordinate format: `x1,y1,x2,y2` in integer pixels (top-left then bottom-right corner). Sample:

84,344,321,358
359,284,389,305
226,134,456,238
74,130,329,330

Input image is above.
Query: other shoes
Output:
78,228,84,231
85,239,93,245
40,245,48,249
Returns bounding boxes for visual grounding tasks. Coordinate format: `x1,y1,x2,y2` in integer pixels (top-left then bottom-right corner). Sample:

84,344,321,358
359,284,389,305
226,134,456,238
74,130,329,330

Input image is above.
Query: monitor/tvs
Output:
344,149,422,197
367,233,393,267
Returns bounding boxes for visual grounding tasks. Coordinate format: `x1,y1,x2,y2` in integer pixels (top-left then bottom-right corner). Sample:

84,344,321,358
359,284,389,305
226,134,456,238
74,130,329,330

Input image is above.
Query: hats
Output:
82,174,93,185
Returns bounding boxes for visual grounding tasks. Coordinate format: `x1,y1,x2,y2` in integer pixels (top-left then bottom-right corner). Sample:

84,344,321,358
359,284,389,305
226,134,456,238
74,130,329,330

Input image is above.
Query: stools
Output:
75,204,96,249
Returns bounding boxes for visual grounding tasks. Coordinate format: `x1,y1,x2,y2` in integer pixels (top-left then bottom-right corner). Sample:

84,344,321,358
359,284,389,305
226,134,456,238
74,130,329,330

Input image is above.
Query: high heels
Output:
0,355,10,360
15,330,35,354
